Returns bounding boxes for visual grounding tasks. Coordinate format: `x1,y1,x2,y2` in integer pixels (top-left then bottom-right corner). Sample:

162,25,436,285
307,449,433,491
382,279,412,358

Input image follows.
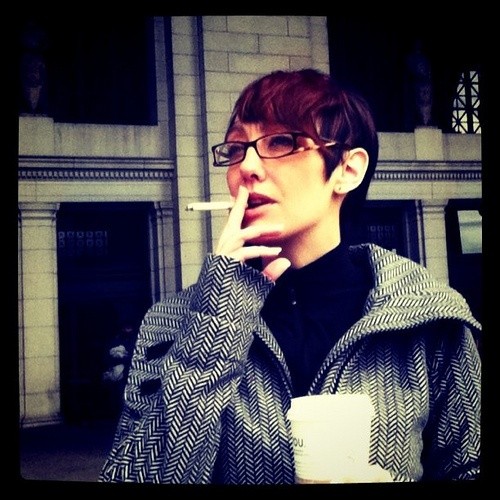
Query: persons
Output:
97,66,485,484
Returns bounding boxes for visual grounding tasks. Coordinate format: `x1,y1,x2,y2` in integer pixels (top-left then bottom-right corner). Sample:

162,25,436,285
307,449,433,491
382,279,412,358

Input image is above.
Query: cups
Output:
286,389,375,483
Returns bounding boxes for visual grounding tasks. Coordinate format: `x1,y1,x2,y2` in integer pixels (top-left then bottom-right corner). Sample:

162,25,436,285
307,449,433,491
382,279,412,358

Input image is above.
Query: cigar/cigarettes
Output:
184,201,247,211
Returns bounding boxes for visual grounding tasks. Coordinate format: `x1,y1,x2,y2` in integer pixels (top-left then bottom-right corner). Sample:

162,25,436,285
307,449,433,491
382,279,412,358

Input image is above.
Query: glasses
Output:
210,133,339,165
